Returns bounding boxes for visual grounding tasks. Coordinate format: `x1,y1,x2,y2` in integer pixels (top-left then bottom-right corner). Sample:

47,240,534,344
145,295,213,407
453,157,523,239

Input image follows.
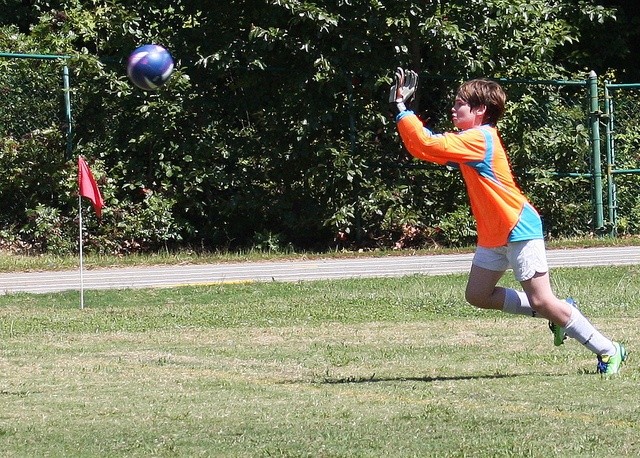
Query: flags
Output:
78,156,104,219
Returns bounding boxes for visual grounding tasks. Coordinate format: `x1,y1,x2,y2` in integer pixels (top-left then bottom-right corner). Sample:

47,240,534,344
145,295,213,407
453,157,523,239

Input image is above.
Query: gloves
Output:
388,67,408,116
404,69,418,111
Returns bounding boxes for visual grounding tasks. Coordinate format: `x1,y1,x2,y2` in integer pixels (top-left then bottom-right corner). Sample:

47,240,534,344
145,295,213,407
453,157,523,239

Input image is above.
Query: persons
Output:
382,67,629,377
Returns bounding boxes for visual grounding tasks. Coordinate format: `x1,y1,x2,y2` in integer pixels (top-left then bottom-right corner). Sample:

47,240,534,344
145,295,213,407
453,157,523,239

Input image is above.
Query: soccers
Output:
127,45,175,91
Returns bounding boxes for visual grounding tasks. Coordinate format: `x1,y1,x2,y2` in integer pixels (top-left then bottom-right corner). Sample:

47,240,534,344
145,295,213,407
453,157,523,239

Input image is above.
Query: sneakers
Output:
596,341,628,378
548,298,579,346
